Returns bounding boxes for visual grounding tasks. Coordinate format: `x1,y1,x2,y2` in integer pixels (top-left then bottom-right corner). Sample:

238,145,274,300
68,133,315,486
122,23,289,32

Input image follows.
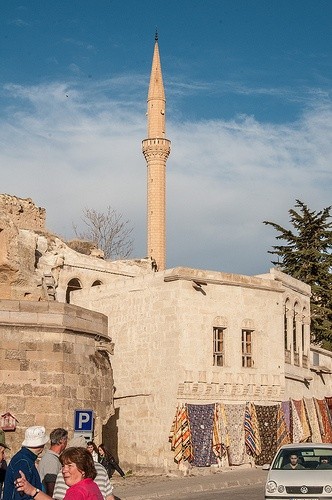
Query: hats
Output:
0,429,12,451
21,425,52,447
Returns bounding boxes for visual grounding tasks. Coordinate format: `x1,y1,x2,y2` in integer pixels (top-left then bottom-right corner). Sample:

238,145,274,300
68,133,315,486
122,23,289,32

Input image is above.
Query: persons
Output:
98,444,126,481
86,441,99,463
36,428,69,496
281,452,306,469
316,456,331,470
13,446,104,500
1,428,11,500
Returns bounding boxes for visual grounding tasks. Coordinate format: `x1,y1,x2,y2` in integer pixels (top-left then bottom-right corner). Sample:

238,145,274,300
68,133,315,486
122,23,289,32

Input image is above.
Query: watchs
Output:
31,488,41,499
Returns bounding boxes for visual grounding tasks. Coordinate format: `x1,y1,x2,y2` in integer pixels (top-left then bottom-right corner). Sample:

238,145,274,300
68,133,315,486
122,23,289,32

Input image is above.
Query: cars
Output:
262,443,332,499
